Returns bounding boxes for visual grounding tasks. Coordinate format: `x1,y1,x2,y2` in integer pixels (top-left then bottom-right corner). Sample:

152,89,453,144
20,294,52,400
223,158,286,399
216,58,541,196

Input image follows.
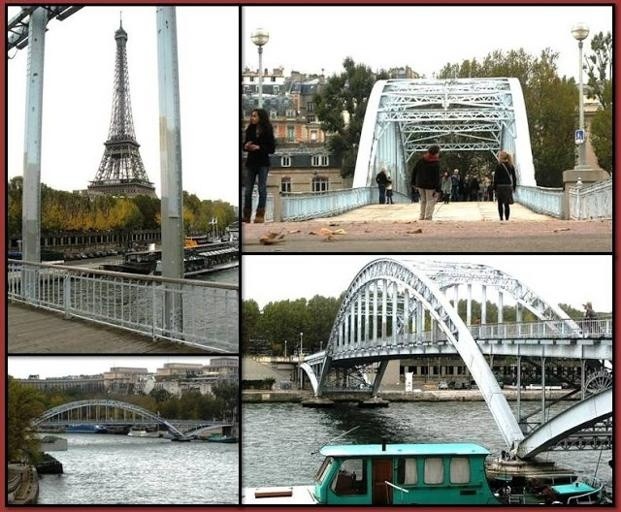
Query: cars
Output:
64,248,117,260
435,377,504,389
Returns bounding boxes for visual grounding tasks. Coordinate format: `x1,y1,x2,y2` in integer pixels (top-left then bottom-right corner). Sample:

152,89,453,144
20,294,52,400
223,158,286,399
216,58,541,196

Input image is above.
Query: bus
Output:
185,236,208,247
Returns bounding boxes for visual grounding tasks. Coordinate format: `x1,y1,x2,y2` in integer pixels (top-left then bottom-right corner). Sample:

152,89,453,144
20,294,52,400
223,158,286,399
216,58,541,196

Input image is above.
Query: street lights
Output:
209,216,219,240
571,23,591,170
318,341,323,352
250,27,270,112
283,340,286,359
298,332,302,356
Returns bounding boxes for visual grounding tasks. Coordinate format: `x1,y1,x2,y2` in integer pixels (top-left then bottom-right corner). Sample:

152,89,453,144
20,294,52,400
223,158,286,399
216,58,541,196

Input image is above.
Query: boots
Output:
254,208,265,223
242,208,252,223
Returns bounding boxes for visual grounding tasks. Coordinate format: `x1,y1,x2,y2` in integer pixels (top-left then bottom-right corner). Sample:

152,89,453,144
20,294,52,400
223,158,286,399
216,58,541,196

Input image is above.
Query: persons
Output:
410,166,494,203
376,166,392,204
385,176,394,204
492,151,517,223
410,144,444,221
240,108,275,225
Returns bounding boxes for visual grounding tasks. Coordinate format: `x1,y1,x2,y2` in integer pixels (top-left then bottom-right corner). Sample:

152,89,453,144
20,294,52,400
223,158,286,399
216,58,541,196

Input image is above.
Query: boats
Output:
101,241,239,279
348,396,389,408
243,443,605,504
127,424,167,437
171,434,195,442
95,422,132,434
206,433,237,443
